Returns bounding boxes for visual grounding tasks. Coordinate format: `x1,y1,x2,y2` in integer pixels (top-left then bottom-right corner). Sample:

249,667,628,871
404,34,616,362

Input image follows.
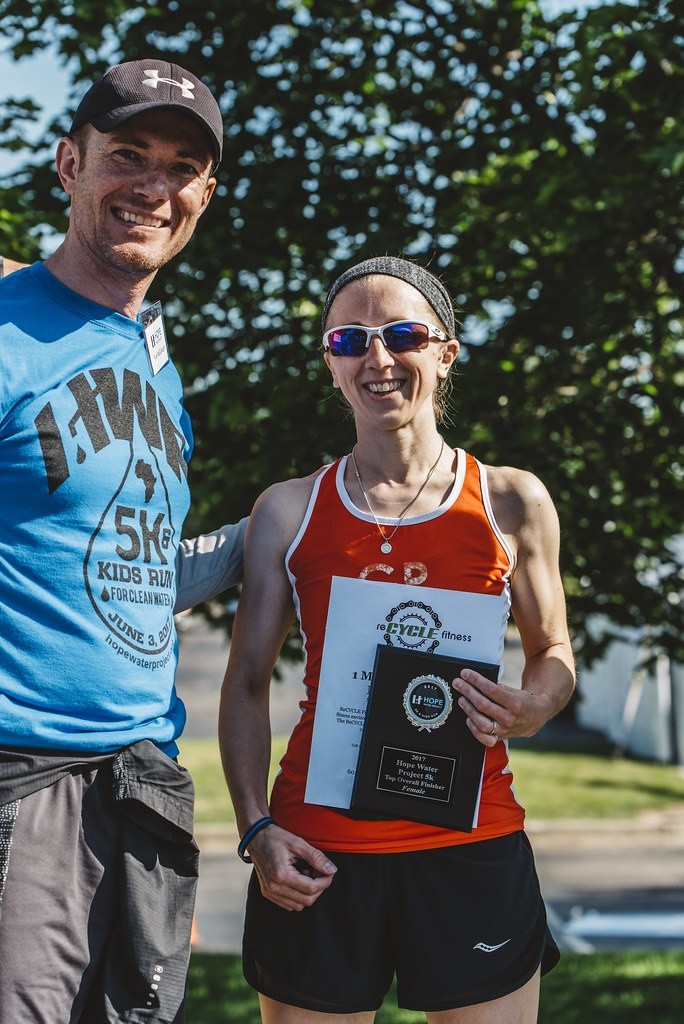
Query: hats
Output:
68,58,224,177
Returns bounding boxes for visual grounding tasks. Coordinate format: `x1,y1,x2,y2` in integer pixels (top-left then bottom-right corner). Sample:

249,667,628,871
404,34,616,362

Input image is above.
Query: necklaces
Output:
351,436,446,553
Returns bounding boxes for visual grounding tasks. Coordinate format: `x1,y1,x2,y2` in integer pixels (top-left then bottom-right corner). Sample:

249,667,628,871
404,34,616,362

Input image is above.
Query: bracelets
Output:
238,817,274,864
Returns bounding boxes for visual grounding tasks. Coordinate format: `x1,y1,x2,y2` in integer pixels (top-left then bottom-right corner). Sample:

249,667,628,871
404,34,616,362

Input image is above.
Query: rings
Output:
490,722,497,735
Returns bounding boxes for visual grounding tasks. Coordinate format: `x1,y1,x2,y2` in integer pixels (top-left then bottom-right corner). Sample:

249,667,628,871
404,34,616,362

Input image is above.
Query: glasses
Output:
319,320,451,356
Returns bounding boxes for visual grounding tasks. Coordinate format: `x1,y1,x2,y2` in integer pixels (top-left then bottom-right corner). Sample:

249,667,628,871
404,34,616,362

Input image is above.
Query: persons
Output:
218,255,577,1023
0,60,333,1024
